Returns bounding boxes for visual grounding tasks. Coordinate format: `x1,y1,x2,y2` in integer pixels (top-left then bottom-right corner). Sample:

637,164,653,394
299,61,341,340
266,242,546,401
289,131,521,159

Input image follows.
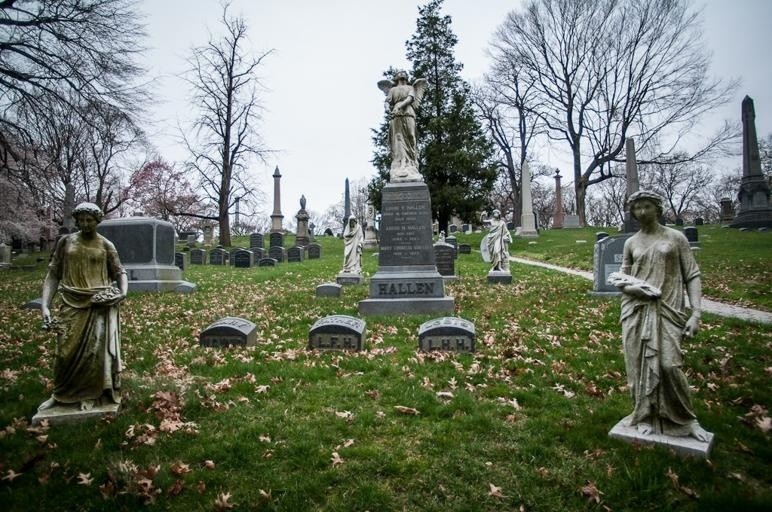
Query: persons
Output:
480,208,514,273
611,189,711,441
36,202,128,413
377,69,429,183
340,214,362,275
